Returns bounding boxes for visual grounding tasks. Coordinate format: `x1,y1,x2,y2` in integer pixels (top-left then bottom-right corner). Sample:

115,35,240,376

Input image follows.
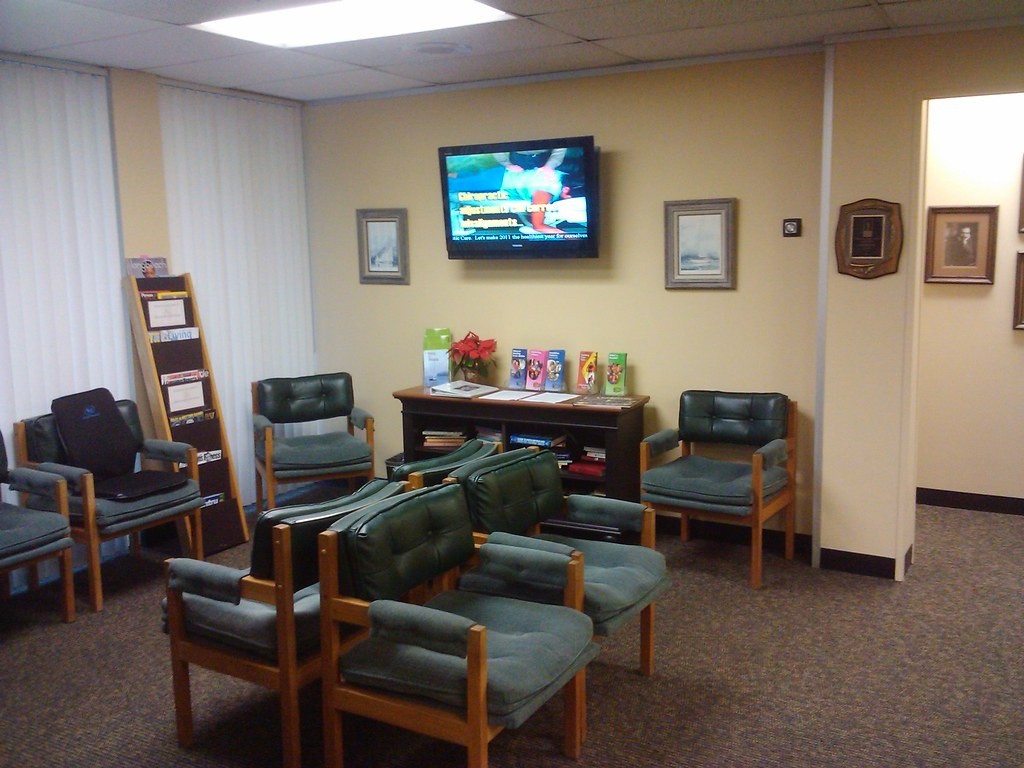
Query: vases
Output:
461,366,482,383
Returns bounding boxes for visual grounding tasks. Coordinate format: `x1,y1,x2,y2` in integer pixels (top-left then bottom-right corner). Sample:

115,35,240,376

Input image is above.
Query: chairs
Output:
0,372,797,768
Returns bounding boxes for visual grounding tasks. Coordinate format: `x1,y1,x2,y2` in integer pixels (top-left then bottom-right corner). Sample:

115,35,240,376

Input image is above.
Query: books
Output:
422,431,469,447
180,450,222,468
149,327,199,343
198,493,224,508
162,369,209,385
126,257,169,278
170,409,215,426
569,462,605,476
140,290,189,299
581,447,606,462
510,432,572,469
430,380,499,398
476,427,501,442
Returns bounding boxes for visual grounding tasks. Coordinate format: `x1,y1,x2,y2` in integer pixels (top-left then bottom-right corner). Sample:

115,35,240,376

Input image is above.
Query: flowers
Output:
445,331,497,378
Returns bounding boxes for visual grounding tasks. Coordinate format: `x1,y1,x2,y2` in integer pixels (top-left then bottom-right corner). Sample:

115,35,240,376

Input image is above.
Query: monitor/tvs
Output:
438,136,599,260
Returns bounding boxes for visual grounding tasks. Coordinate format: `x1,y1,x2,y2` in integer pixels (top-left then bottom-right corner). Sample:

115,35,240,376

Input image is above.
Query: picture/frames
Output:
835,198,903,279
662,198,738,290
357,209,410,284
923,206,998,284
1013,251,1024,329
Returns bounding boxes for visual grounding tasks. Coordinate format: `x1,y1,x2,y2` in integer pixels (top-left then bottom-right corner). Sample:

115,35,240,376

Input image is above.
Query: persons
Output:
492,148,567,233
948,226,974,265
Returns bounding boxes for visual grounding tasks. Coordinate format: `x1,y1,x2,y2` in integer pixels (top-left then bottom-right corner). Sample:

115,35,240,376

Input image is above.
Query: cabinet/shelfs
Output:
392,385,651,504
